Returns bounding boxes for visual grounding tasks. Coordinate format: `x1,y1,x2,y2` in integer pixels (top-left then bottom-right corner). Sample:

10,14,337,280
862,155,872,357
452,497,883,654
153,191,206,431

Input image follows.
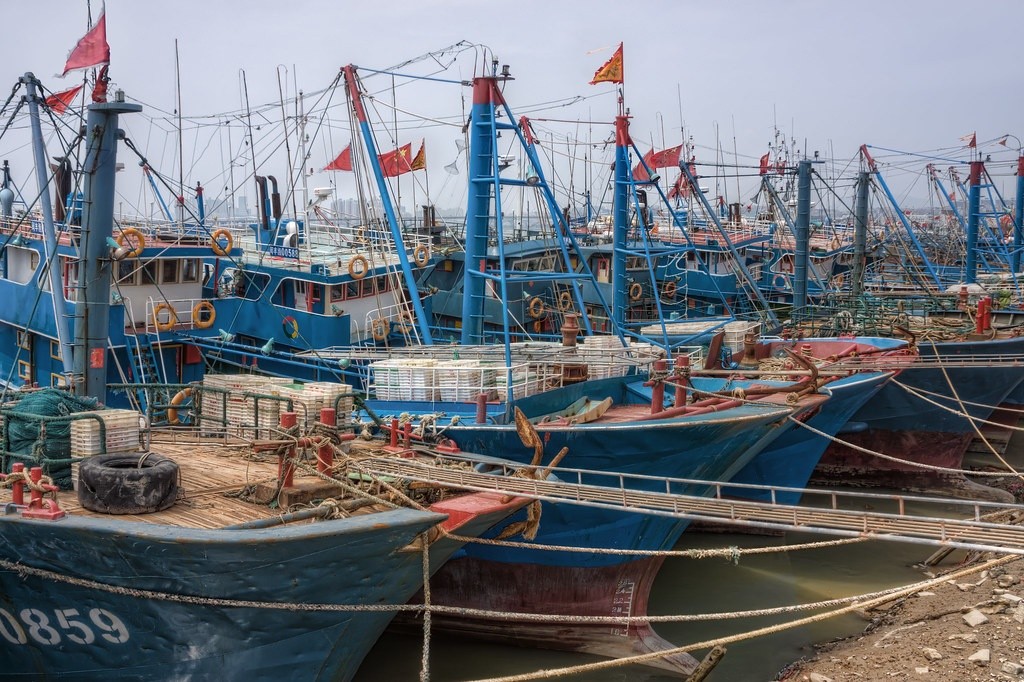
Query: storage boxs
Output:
200,374,352,440
368,358,537,402
584,320,748,380
69,409,139,491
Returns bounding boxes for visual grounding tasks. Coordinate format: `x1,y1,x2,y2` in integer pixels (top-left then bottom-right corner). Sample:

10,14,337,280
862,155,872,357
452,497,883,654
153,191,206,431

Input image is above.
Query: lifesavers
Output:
117,228,145,258
77,451,180,515
879,230,885,241
560,292,571,310
372,318,389,341
358,226,370,243
212,229,233,256
193,302,216,329
347,255,369,280
152,303,176,331
666,282,676,297
630,284,642,300
831,235,839,251
169,388,201,427
414,245,429,267
397,311,413,334
772,274,787,290
530,298,544,318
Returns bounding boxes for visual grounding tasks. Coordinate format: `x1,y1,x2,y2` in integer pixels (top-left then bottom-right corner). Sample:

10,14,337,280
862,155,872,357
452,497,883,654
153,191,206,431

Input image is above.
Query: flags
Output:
46,86,83,115
650,145,681,168
410,145,426,170
760,154,769,174
969,134,976,147
588,43,625,85
769,162,785,176
62,14,110,77
378,143,410,177
633,150,656,182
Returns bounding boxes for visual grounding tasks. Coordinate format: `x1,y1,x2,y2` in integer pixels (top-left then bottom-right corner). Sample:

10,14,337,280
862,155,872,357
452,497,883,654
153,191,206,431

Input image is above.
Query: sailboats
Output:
0,1,1024,682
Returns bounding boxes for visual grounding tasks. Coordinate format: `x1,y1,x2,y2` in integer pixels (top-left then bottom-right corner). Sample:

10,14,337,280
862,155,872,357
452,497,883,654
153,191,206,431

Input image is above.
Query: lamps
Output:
12,232,29,248
527,165,539,184
647,167,660,182
339,357,348,369
262,337,274,356
1003,207,1012,214
113,291,122,304
429,285,438,294
809,221,823,236
219,329,234,342
101,239,130,267
523,291,531,300
675,275,681,282
331,305,344,316
